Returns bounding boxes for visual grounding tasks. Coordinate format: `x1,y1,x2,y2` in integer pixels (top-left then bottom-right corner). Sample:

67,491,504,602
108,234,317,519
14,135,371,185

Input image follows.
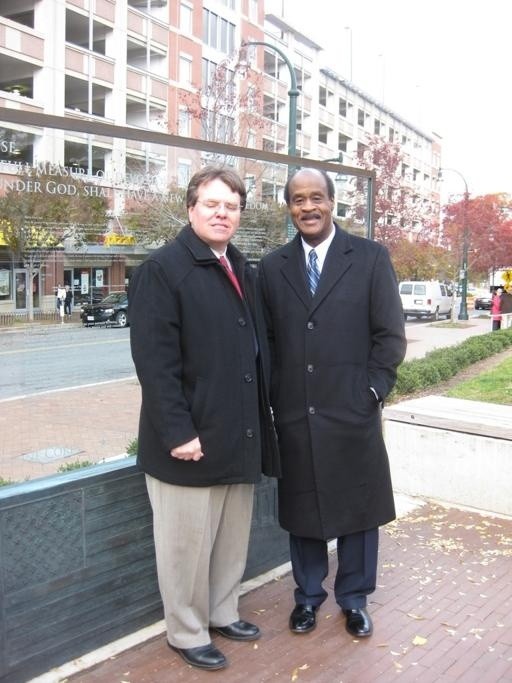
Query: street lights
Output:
237,41,302,177
436,166,468,320
412,222,440,282
320,150,347,183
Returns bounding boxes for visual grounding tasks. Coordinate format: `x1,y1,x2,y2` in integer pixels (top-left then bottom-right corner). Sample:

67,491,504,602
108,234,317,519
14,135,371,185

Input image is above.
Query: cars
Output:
448,281,478,298
77,289,128,328
474,293,493,309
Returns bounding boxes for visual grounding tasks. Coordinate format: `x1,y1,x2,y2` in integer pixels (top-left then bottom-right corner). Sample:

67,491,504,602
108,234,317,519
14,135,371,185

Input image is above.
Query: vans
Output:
397,281,455,322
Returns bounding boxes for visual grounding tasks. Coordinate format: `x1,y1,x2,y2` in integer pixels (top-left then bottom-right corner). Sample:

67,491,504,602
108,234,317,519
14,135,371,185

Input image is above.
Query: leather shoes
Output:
341,607,373,637
209,620,260,641
167,640,227,671
289,604,320,633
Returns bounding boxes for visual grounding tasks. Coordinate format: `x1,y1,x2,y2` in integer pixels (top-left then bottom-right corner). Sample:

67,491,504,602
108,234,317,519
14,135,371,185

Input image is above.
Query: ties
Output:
306,251,321,298
218,256,243,300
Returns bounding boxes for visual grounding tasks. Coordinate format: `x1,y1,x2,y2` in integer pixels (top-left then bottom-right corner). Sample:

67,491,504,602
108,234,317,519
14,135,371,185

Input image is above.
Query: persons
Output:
56,284,72,318
256,167,407,636
127,165,272,671
492,287,504,331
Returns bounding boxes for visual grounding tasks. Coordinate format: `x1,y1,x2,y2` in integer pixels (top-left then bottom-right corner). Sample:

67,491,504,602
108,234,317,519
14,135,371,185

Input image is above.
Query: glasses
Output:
196,199,244,210
